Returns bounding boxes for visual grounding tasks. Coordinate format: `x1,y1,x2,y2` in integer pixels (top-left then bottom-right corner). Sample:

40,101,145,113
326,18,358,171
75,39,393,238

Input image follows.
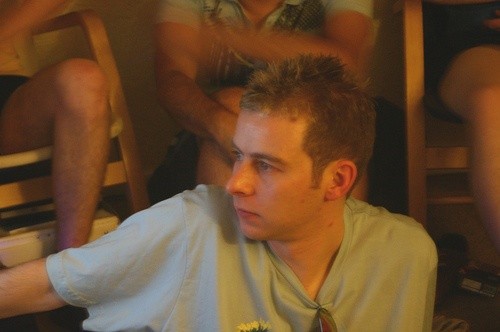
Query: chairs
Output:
0,12,150,270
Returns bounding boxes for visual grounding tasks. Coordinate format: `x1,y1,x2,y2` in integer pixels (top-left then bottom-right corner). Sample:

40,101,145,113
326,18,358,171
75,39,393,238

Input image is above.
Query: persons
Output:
0,52,439,331
0,0,112,272
424,0,500,289
152,0,378,193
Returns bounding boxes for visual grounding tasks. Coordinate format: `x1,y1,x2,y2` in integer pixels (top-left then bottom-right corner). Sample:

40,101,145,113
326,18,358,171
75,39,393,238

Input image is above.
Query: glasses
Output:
316,308,337,332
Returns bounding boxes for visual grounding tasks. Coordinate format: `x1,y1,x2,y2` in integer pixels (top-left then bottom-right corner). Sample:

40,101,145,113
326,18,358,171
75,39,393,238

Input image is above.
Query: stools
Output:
376,1,476,245
